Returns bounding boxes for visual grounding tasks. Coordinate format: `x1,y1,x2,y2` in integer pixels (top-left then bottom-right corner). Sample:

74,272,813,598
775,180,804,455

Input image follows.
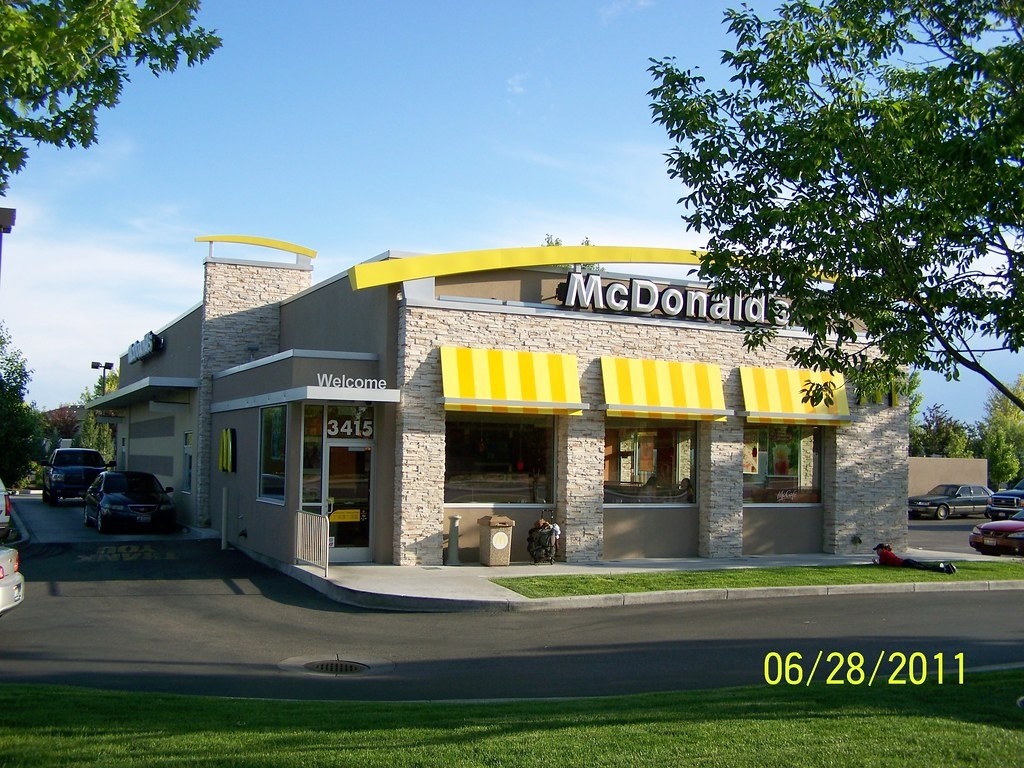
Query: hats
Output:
873,543,885,550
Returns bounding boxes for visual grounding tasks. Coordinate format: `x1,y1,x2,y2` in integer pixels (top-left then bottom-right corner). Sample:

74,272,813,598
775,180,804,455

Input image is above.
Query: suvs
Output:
985,479,1024,521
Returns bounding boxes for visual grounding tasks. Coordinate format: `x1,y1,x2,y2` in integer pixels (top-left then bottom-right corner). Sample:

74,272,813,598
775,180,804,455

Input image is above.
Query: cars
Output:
84,471,178,534
0,545,25,617
42,447,117,503
968,509,1024,556
908,484,995,520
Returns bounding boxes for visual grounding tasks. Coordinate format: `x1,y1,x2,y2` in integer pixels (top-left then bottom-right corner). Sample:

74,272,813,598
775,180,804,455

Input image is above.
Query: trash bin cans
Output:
477,515,516,567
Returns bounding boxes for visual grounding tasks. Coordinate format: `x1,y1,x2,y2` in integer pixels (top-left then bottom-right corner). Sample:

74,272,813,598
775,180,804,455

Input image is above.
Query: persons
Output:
870,543,956,575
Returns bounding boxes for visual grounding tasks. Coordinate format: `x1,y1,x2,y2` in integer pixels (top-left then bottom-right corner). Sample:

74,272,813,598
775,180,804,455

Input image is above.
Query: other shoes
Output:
945,564,957,573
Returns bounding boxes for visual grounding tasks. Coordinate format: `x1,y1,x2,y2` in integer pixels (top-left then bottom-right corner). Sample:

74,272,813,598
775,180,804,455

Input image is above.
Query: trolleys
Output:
534,509,556,565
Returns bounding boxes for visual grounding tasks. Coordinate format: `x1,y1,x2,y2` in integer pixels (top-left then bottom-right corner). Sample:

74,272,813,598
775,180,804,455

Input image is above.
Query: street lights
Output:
91,362,113,396
